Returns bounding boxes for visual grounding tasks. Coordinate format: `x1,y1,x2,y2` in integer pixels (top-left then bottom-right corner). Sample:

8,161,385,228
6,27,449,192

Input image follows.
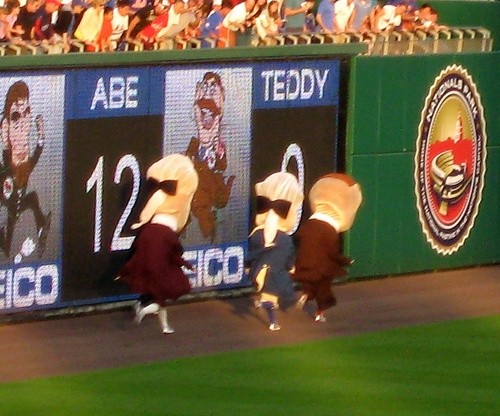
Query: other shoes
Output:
269,323,279,331
162,326,176,334
298,295,306,309
253,297,261,309
315,313,327,322
134,299,146,322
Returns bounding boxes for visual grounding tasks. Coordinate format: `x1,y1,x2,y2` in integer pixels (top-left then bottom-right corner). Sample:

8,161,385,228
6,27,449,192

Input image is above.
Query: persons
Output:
0,0,451,58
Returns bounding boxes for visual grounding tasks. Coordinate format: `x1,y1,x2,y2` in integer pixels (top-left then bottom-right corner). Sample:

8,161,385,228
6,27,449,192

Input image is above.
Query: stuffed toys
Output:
234,172,304,331
290,173,365,323
119,153,198,333
